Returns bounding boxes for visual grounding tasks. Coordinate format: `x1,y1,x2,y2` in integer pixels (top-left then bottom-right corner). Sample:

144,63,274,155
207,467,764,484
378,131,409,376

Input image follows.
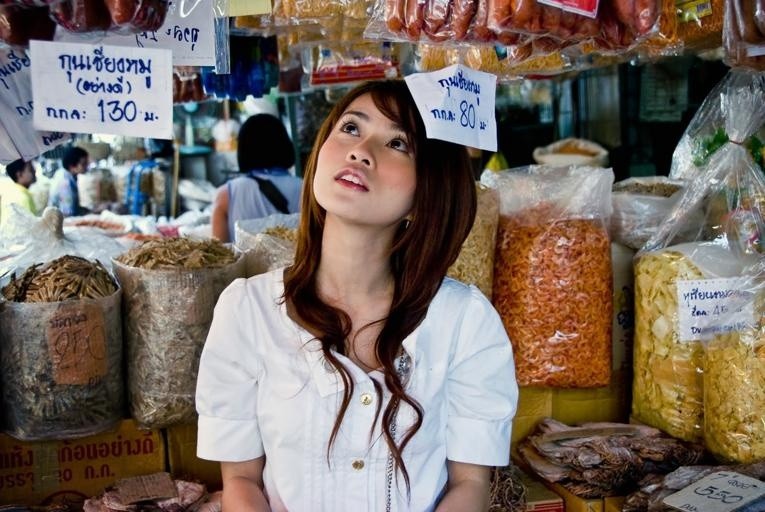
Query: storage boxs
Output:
1,416,222,511
495,464,565,512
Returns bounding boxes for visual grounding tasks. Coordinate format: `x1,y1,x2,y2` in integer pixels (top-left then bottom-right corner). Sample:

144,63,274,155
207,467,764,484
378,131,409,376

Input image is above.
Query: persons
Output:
46,145,99,221
193,77,519,511
0,157,40,235
210,112,303,243
